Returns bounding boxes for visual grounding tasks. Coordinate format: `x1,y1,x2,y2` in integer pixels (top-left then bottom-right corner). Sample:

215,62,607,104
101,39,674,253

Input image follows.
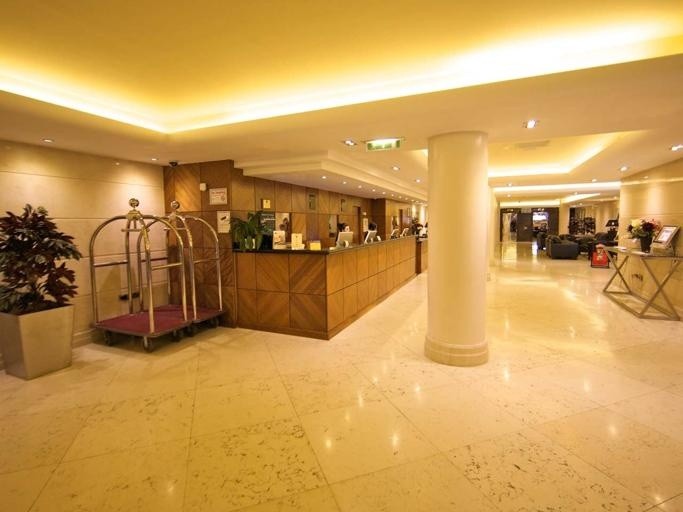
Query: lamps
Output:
604,220,618,241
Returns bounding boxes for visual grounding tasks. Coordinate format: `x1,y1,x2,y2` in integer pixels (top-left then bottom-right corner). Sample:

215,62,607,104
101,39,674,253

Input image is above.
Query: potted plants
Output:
227,209,272,252
0,203,84,381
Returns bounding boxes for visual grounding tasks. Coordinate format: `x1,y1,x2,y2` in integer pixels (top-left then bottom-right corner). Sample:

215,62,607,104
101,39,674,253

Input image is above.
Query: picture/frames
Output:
653,225,680,245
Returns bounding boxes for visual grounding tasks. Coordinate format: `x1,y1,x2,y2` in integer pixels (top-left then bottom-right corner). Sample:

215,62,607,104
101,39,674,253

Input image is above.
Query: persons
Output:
337,223,350,232
364,222,378,241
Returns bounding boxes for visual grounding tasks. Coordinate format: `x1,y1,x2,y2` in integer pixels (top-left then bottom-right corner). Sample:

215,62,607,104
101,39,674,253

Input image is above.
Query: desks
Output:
601,245,683,321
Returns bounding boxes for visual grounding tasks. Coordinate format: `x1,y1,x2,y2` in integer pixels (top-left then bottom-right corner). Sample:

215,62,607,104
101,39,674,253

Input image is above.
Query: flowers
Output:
627,217,662,240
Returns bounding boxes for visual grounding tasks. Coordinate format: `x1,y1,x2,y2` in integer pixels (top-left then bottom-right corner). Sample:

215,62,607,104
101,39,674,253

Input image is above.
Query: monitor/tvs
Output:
401,228,409,236
650,225,680,249
390,229,399,238
364,231,377,243
336,232,353,247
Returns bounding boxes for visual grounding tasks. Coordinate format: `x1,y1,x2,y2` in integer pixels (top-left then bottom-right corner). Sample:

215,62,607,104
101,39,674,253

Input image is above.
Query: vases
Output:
640,237,651,253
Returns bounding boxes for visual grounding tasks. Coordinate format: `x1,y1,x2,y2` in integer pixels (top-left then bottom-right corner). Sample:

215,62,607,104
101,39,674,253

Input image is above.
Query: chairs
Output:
544,235,579,259
